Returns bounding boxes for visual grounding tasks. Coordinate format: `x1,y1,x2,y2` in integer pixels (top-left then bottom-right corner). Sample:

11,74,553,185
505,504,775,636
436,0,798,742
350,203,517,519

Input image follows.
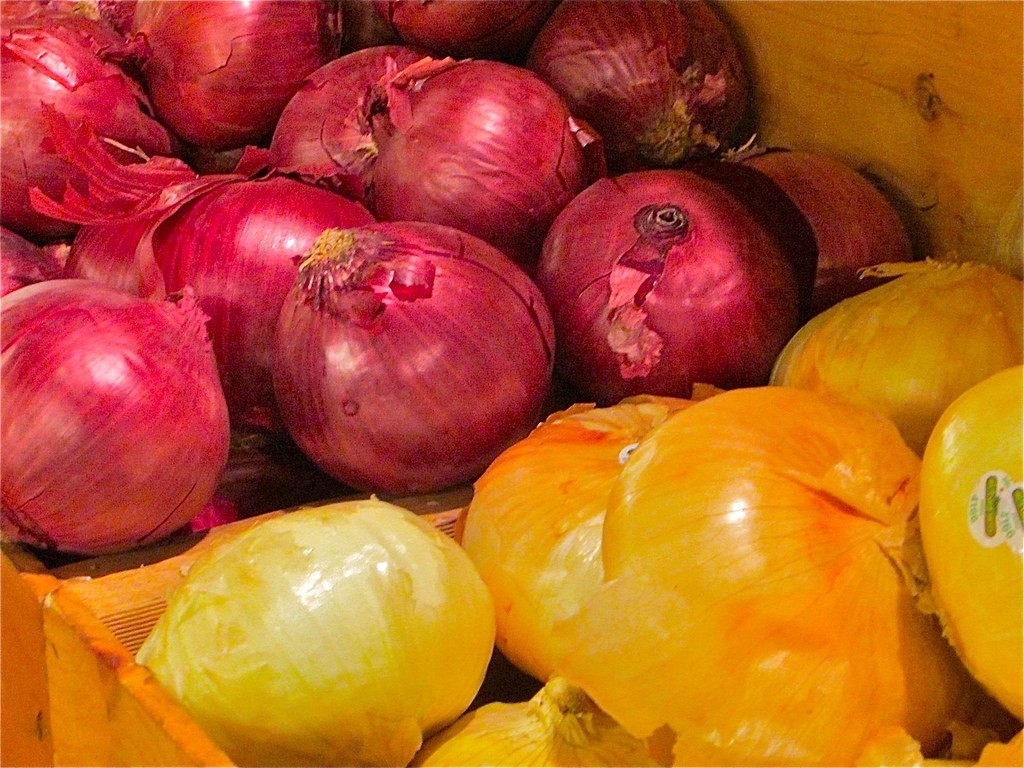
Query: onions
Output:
0,0,1024,768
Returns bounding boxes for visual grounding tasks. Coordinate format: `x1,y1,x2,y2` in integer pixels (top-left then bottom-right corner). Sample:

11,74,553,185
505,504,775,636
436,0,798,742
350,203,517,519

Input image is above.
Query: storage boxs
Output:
0,488,480,768
